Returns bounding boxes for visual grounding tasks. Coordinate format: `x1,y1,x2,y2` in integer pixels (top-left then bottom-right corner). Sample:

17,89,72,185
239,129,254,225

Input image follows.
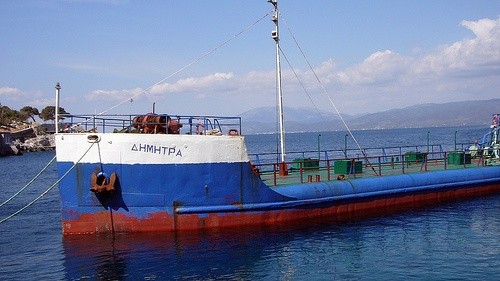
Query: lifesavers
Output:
196,121,204,134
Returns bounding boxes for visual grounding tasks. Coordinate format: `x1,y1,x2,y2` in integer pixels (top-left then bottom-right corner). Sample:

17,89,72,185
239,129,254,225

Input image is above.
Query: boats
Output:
54,1,500,236
